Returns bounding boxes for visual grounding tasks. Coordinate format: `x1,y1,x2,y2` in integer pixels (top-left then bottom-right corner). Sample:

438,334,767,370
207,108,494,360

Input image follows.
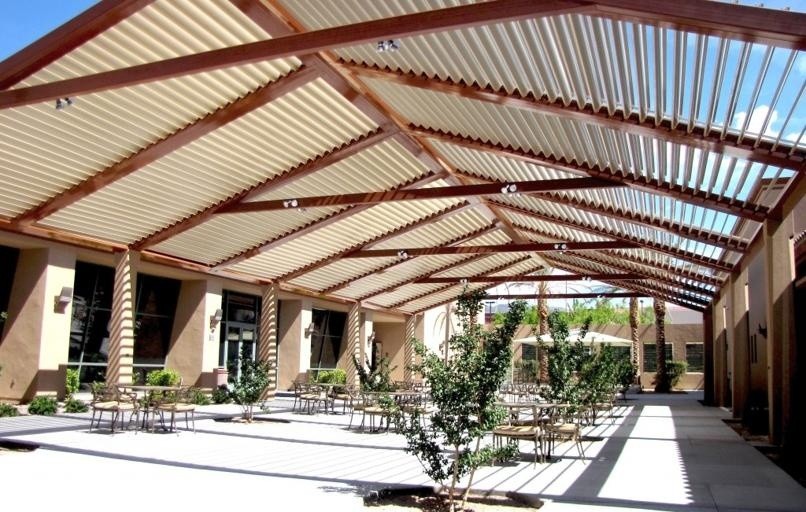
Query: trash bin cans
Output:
213,368,228,390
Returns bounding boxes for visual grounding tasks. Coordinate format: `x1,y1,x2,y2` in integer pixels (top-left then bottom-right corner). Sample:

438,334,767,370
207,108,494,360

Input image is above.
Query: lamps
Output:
283,198,299,209
596,293,606,301
367,331,376,348
210,309,223,332
554,243,568,249
54,286,71,314
55,98,72,110
376,39,400,53
497,296,505,300
755,321,767,339
459,277,468,282
582,274,592,281
305,322,315,338
397,250,409,260
500,184,516,193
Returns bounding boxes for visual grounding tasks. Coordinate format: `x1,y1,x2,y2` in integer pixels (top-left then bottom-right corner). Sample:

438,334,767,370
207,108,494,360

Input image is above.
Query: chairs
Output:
291,382,624,470
87,381,195,438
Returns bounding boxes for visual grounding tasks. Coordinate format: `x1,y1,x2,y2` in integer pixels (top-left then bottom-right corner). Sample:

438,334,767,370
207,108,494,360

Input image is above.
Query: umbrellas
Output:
509,324,638,362
